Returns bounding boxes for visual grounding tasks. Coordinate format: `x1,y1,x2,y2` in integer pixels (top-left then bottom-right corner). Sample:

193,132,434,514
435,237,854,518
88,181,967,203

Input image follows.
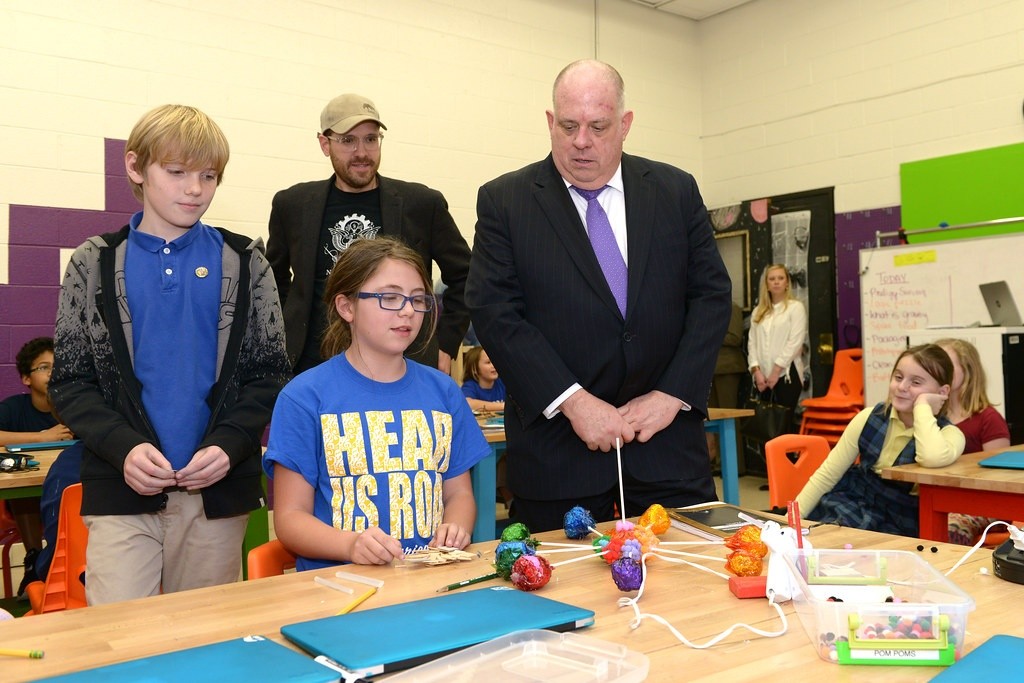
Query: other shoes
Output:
18,549,40,597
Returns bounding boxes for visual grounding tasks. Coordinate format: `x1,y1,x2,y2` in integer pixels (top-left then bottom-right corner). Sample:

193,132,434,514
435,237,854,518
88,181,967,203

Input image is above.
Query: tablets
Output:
6,440,79,453
979,451,1024,470
29,586,595,683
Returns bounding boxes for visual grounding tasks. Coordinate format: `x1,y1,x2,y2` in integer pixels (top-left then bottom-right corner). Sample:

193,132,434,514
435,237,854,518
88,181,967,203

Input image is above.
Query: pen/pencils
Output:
437,573,500,593
334,586,379,614
0,647,45,659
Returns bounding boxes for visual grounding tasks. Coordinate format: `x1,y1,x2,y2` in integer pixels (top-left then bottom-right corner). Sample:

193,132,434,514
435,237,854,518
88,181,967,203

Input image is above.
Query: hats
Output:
317,93,387,139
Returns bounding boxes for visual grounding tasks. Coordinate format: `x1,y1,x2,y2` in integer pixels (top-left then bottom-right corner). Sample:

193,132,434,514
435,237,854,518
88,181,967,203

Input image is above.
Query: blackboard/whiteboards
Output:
857,232,1024,420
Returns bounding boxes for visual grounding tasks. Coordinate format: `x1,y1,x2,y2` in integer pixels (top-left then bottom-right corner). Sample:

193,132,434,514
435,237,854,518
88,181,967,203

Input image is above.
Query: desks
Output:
0,504,1024,683
0,403,756,512
882,443,1024,548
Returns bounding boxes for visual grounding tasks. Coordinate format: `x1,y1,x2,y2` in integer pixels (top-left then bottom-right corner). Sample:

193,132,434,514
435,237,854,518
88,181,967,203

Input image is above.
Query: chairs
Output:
247,539,303,583
23,483,91,617
0,499,45,599
766,349,864,519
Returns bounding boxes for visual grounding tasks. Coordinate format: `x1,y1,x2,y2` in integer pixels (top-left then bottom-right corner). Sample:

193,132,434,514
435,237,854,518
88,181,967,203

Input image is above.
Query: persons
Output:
0,337,81,600
936,338,1013,547
706,303,748,478
748,264,806,490
48,105,290,607
262,239,493,572
786,343,965,538
466,61,730,533
266,96,472,379
463,347,514,507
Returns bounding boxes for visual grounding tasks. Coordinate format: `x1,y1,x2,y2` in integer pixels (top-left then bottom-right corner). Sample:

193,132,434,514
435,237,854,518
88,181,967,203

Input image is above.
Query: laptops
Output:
979,281,1023,326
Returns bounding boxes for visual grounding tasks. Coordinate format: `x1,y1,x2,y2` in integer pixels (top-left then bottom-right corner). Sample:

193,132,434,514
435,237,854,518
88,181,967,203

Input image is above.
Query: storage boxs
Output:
777,546,974,666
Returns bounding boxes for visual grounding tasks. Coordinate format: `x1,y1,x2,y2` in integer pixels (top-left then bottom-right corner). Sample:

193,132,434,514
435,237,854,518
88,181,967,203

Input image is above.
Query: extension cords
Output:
992,527,1024,585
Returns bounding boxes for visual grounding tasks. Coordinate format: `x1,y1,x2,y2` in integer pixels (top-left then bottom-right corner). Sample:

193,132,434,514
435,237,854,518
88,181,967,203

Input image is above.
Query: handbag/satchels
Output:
744,383,791,456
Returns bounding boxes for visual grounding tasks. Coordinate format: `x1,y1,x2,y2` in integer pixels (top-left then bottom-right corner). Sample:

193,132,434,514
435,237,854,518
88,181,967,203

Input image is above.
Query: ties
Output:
570,185,628,318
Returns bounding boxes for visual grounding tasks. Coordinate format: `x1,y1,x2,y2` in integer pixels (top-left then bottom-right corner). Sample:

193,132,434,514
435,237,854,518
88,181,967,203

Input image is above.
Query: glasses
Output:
28,364,54,373
355,293,435,312
327,133,384,151
